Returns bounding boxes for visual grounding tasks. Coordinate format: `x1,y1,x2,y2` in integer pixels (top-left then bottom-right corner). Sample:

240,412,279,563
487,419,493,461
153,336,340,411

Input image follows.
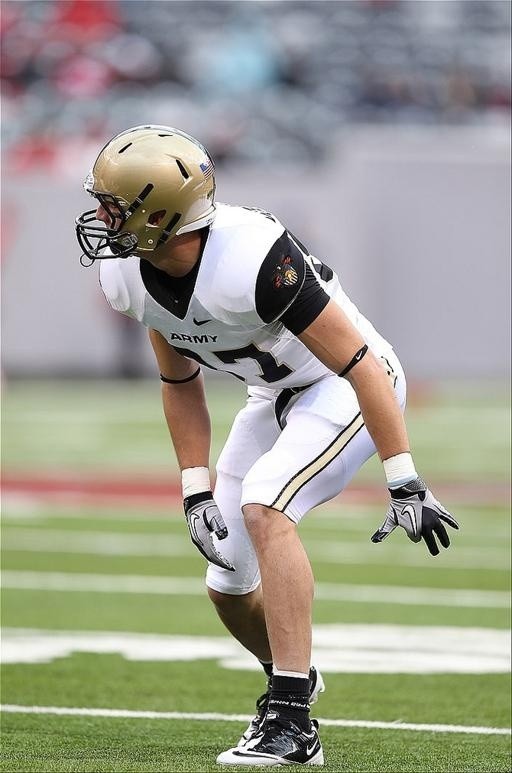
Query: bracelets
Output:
382,450,418,486
181,466,212,497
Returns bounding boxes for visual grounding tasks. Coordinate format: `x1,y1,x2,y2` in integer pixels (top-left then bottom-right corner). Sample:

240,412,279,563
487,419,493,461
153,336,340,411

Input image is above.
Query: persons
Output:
75,126,461,768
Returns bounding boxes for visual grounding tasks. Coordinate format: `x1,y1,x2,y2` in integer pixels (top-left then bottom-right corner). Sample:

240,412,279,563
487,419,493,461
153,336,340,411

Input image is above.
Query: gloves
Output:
371,475,459,556
183,491,235,572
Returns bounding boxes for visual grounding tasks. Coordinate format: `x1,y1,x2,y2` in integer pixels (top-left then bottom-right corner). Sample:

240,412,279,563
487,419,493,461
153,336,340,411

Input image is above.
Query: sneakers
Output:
215,709,324,768
236,665,326,748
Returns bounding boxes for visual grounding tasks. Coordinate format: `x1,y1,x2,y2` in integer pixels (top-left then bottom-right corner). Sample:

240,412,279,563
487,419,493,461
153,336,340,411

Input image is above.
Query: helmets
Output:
75,125,217,268
